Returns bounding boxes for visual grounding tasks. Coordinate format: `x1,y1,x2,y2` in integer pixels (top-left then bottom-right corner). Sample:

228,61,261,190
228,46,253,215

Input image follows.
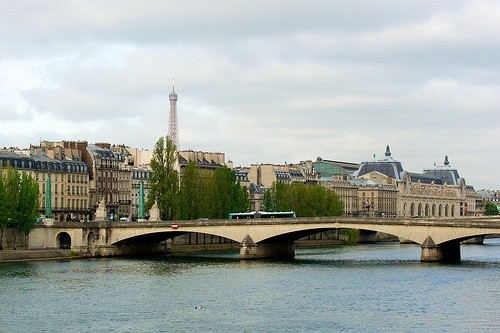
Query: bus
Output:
229,211,296,220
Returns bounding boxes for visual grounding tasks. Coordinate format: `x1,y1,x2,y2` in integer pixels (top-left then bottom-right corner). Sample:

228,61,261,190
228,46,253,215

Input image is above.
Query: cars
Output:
107,212,150,221
196,218,209,221
